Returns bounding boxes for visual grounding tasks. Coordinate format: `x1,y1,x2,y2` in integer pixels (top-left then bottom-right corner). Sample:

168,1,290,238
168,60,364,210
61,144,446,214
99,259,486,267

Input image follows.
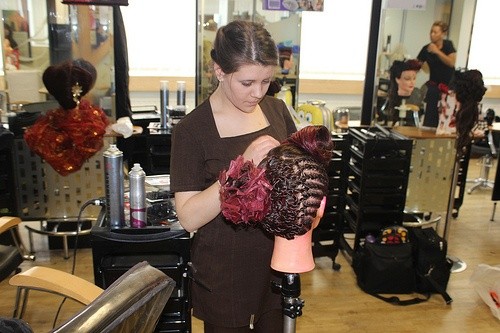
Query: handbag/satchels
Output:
353,225,454,305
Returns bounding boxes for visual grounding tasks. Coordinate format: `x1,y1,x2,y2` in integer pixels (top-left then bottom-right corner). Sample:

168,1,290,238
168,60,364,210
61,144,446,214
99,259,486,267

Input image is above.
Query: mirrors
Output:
360,0,476,133
195,0,303,112
0,0,114,128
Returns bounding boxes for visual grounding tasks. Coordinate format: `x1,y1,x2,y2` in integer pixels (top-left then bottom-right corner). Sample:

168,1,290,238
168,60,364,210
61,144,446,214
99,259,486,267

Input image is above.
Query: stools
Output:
465,143,497,194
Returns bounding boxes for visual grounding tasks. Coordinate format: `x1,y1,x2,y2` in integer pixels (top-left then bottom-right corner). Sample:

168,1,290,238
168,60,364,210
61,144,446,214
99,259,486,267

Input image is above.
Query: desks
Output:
399,126,458,242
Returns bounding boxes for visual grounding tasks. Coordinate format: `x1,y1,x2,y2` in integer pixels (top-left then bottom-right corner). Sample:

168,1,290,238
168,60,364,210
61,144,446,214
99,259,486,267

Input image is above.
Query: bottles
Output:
103,143,125,228
332,109,349,134
128,164,147,228
160,81,169,130
176,81,186,105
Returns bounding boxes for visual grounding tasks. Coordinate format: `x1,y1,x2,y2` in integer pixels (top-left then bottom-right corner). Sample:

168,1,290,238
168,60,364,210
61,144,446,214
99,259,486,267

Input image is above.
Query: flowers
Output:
218,155,270,224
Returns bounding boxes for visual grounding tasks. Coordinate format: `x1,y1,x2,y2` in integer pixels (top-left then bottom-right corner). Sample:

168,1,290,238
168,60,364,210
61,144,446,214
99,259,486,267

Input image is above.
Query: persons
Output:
220,125,334,273
278,47,295,75
96,26,107,47
450,69,488,146
388,59,421,96
169,20,298,333
42,58,98,122
417,21,457,127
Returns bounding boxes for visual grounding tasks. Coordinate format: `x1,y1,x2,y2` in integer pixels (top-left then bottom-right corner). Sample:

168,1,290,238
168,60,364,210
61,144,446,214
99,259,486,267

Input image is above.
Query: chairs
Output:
0,260,177,333
